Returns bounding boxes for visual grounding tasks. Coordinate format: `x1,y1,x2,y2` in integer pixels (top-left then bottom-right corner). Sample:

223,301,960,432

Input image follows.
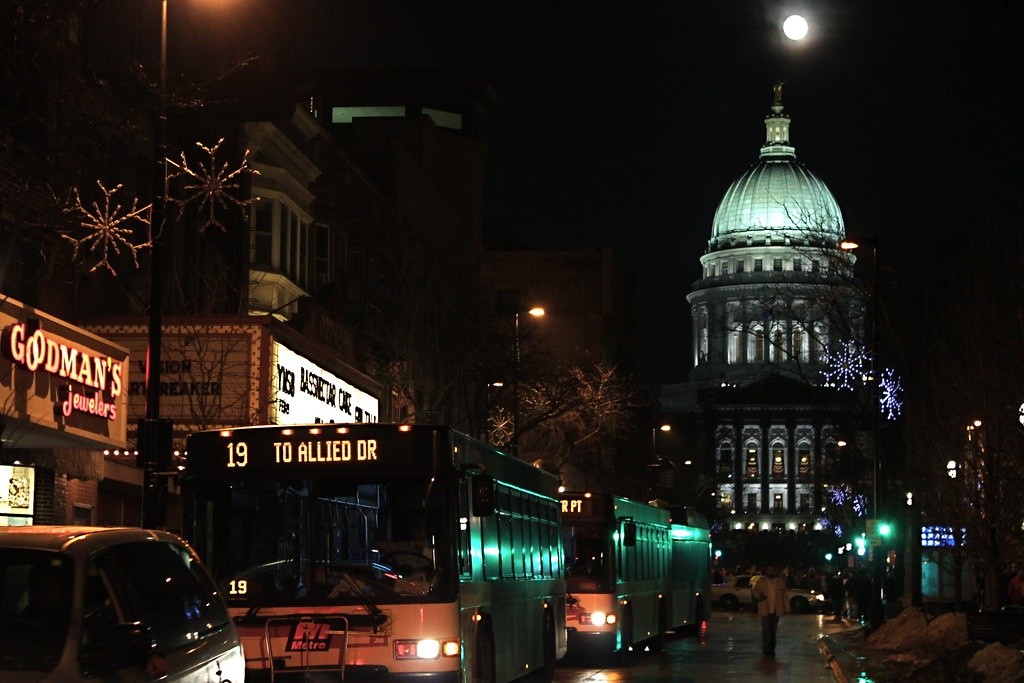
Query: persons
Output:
1008,566,1024,605
713,563,872,656
374,513,436,580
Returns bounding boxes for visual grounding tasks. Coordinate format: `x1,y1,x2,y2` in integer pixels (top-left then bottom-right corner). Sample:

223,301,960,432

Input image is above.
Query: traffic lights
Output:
852,535,867,557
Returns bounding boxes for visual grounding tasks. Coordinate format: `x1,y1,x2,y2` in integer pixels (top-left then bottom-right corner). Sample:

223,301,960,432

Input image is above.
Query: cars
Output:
712,574,824,615
0,525,246,683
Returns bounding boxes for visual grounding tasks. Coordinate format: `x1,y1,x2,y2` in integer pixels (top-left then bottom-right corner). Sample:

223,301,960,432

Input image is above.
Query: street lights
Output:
145,1,236,420
512,305,546,456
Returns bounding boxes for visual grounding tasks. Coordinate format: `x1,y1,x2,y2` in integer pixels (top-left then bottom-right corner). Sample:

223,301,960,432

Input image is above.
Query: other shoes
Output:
832,619,841,623
763,649,775,656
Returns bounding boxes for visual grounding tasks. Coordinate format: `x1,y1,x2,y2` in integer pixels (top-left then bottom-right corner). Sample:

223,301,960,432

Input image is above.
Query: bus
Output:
145,424,568,683
664,504,712,635
558,491,674,663
